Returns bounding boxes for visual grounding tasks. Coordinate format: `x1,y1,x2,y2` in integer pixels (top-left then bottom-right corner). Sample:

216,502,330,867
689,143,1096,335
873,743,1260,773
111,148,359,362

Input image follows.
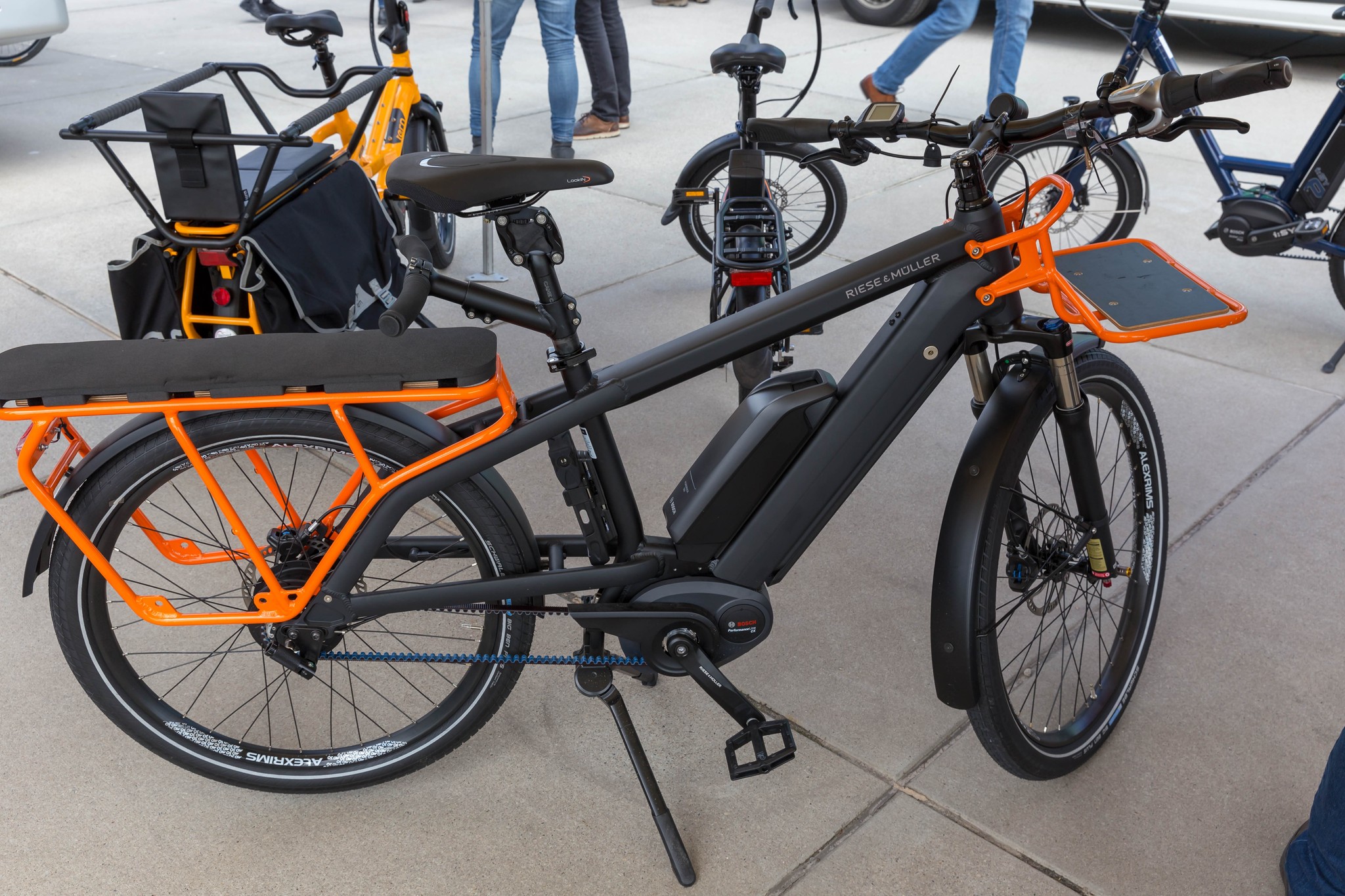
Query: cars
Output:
841,0,1343,40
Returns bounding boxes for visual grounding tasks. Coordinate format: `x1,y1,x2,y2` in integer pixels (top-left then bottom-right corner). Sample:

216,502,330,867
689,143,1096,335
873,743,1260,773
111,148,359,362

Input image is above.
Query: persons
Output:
573,0,632,141
466,0,579,160
859,0,1036,157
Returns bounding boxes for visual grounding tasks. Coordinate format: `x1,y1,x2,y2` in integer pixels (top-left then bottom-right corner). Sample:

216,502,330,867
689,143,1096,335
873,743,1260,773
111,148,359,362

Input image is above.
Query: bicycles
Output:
980,0,1344,377
55,1,456,341
661,1,847,411
0,55,1297,887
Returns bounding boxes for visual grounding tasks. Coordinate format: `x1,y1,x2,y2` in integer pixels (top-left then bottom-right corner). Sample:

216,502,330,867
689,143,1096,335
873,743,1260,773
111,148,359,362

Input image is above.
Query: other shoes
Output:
551,139,575,159
470,136,494,154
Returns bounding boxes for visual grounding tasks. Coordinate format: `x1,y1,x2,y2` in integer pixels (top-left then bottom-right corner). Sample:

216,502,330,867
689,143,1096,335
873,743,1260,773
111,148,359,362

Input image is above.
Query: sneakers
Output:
618,115,629,129
572,112,620,140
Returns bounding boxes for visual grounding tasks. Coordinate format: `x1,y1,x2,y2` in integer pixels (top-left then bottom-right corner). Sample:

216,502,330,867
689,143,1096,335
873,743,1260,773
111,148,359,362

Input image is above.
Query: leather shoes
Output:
859,73,909,122
